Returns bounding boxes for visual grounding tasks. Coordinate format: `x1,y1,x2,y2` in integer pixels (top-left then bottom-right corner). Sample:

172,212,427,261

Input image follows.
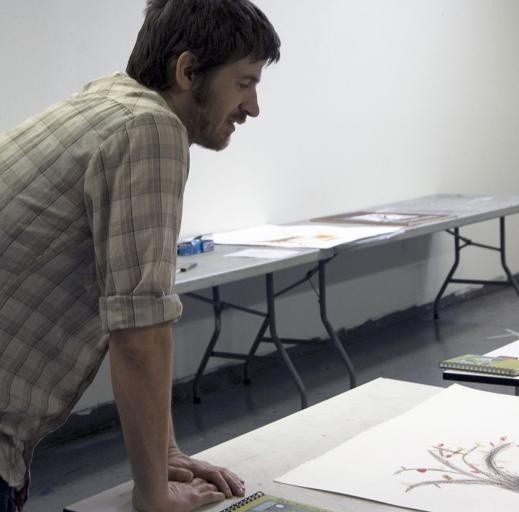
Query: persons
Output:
1,0,285,512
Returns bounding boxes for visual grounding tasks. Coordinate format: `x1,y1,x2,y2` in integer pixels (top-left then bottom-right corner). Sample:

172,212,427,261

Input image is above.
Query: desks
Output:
443,338,519,396
66,377,519,512
170,244,320,411
214,192,518,388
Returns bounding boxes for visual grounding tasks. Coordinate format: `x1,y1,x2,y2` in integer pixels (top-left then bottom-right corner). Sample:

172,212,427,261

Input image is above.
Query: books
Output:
436,352,519,378
219,489,340,512
